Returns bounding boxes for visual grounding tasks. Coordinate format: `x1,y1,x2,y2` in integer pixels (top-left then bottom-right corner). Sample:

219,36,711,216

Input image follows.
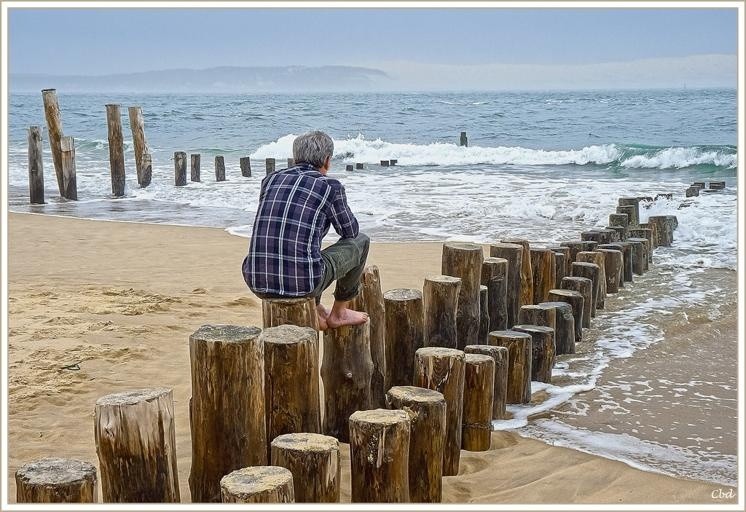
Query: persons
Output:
242,130,370,330
